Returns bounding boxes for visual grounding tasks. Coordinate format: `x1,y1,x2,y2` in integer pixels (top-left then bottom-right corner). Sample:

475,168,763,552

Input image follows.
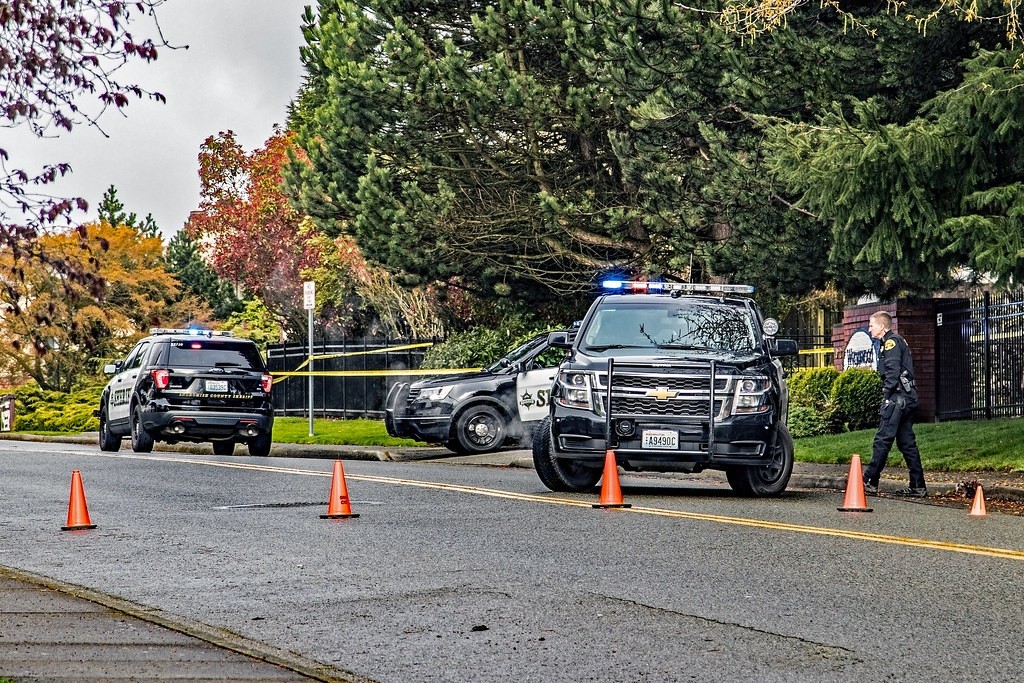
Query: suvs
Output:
533,281,795,497
93,326,275,456
384,319,582,454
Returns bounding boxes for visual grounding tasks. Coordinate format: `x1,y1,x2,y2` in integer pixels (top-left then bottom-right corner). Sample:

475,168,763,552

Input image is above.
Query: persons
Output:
845,311,928,497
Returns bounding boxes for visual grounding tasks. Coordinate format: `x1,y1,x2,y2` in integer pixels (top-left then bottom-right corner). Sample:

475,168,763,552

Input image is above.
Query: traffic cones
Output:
591,449,632,508
319,461,360,519
837,455,874,512
968,485,989,516
60,471,97,531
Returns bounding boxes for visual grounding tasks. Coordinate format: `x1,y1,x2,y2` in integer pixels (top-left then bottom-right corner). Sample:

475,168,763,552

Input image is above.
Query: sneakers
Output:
897,487,928,498
844,477,879,493
